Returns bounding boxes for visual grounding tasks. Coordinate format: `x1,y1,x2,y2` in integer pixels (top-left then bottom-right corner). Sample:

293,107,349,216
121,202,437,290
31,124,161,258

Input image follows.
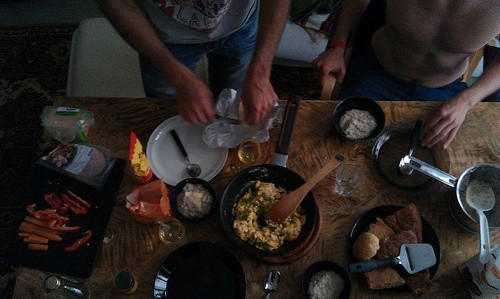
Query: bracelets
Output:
325,38,346,51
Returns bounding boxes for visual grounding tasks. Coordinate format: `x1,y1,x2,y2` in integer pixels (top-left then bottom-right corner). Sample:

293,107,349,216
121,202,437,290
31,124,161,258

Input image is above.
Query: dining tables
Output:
12,96,500,299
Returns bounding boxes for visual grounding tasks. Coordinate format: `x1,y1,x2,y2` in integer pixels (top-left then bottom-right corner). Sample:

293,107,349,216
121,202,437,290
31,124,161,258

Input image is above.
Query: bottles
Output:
41,276,95,298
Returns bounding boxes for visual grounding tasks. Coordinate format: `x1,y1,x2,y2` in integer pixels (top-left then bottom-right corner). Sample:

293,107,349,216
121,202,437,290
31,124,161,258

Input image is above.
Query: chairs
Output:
67,0,386,100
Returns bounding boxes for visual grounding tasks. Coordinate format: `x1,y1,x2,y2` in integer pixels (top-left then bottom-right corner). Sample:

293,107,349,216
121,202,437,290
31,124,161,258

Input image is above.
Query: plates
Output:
145,114,228,186
372,124,449,187
349,204,441,292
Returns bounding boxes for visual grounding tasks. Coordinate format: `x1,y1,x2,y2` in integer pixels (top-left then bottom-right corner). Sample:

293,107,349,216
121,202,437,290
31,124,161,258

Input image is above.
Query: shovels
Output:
349,244,436,276
466,182,495,264
264,154,344,225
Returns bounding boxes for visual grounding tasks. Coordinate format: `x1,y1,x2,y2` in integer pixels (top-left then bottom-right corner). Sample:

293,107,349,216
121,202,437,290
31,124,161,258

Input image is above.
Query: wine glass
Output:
235,133,266,168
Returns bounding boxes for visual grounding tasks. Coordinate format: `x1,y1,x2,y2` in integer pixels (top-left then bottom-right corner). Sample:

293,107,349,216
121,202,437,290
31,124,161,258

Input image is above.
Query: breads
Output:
351,204,430,292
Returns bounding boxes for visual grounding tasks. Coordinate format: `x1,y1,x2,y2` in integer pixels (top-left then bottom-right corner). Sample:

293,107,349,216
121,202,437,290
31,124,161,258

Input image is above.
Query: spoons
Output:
264,270,281,299
399,116,424,178
169,129,201,177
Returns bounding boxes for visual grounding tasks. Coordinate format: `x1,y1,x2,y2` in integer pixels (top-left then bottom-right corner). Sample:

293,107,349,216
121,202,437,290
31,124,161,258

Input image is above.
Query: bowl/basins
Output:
334,96,387,142
172,179,216,220
301,260,349,299
153,240,246,299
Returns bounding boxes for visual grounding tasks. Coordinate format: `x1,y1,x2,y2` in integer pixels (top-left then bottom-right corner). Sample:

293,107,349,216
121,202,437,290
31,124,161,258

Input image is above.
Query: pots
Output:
403,153,500,235
220,94,323,264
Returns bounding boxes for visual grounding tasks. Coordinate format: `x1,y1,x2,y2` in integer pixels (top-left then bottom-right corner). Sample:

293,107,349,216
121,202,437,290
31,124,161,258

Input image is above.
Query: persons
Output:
312,0,500,151
96,0,290,127
